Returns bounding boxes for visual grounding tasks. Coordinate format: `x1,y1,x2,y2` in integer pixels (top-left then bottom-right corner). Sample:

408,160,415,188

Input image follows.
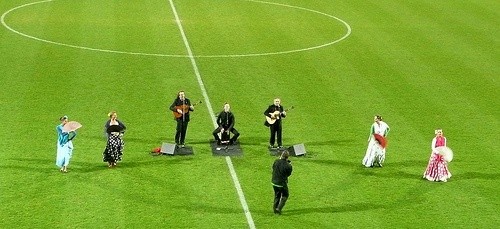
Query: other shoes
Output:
229,139,233,145
182,144,185,148
273,208,281,215
109,165,112,168
277,146,283,148
64,170,68,172
378,161,382,167
270,144,274,148
217,139,221,146
60,169,63,172
370,165,373,167
114,162,117,166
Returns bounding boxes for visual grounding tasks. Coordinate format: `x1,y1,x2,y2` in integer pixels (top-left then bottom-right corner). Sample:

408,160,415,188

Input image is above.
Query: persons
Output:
362,115,391,167
423,129,452,182
264,97,287,148
169,90,194,145
56,114,77,173
271,150,293,215
102,111,126,168
212,102,241,146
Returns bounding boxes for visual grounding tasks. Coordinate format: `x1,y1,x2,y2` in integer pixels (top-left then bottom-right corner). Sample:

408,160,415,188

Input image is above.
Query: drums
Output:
221,130,230,143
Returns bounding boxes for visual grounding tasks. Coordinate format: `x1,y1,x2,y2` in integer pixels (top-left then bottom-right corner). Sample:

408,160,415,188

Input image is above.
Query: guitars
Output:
172,100,202,118
266,106,295,125
